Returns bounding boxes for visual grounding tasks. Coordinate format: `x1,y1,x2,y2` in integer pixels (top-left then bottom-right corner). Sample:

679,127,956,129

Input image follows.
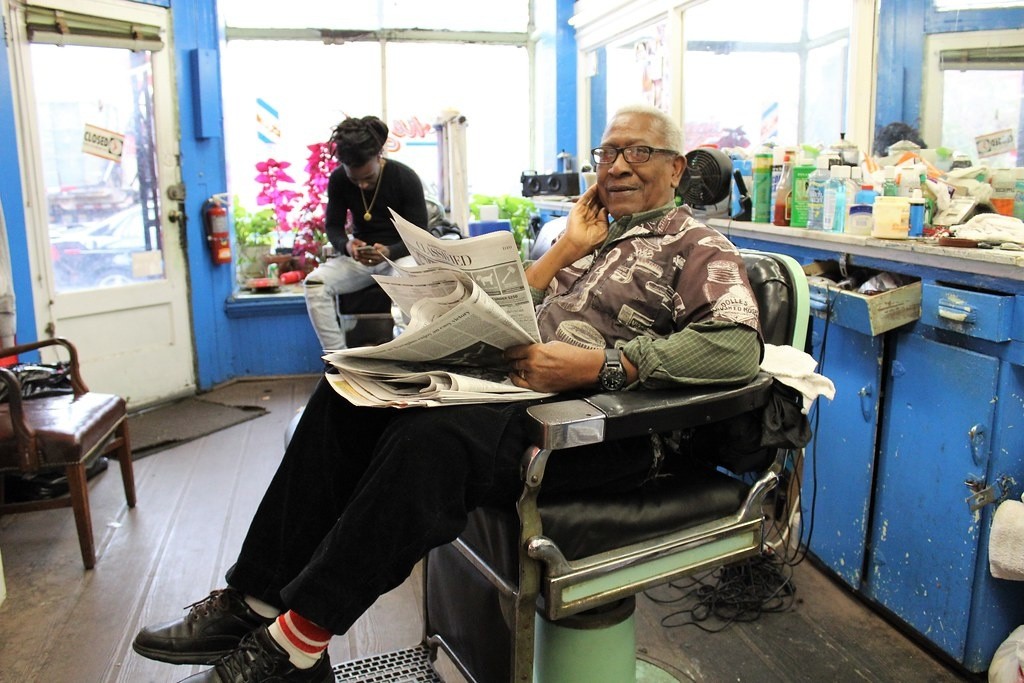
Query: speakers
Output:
521,173,580,198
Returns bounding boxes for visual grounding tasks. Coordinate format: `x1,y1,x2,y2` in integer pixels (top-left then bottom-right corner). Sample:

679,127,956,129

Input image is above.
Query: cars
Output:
46,180,159,291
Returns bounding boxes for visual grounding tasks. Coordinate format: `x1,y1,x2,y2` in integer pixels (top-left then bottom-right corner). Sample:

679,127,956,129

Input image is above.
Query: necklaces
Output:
359,163,382,219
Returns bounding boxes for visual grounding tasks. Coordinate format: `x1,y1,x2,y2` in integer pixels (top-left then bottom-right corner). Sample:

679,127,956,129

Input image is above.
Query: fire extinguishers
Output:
200,192,234,264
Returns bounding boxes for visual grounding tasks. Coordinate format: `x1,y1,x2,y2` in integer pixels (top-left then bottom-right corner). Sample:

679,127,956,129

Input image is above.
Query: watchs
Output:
598,348,628,393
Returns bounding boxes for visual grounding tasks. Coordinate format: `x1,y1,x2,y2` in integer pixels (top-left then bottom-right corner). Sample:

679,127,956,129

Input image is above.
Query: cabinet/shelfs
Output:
794,256,1016,673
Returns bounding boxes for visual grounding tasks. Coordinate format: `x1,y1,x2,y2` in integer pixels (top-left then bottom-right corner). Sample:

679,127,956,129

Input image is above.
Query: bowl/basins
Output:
990,198,1014,216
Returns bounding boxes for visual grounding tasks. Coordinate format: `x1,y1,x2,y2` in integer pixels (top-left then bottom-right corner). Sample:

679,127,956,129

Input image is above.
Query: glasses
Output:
591,145,680,163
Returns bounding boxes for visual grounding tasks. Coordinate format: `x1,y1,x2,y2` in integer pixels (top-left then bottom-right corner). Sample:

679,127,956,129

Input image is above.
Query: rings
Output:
519,370,525,380
368,260,372,264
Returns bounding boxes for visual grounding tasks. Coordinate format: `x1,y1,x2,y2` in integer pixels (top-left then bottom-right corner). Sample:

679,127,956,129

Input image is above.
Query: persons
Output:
133,108,764,683
302,114,428,369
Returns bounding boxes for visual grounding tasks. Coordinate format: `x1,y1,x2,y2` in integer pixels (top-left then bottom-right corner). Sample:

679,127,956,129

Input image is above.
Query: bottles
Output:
770,165,783,224
280,270,305,284
884,178,897,197
753,143,773,223
856,185,879,204
790,159,817,228
773,151,796,226
556,150,571,173
993,169,1014,197
1013,179,1024,223
268,262,279,280
808,159,831,230
823,160,873,239
933,147,950,173
900,167,938,237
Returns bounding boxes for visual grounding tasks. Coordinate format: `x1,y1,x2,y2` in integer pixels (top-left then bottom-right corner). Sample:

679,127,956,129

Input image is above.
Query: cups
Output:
480,205,499,220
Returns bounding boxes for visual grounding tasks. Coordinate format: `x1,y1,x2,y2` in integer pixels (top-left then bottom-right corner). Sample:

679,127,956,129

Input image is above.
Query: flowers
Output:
255,139,351,268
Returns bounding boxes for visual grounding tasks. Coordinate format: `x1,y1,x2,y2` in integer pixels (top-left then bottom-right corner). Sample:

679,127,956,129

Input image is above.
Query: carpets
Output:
95,392,270,464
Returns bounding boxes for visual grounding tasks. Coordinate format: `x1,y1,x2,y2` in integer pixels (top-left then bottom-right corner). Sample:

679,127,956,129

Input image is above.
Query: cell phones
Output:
356,246,375,251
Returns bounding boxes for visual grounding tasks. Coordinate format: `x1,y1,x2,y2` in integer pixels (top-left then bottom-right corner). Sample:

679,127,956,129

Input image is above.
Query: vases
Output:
274,246,303,275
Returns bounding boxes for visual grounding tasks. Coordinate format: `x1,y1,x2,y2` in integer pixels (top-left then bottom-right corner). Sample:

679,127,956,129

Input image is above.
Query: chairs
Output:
0,338,136,568
331,247,826,683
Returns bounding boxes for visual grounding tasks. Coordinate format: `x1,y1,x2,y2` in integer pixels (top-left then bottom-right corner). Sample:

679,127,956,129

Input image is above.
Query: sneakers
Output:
132,589,275,665
176,622,336,683
